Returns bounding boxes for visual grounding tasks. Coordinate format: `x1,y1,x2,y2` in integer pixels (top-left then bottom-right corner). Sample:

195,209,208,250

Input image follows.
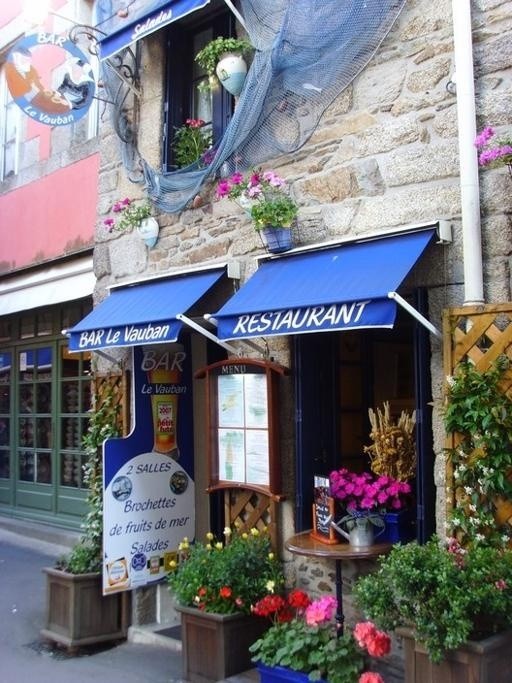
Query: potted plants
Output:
251,196,299,253
40,544,130,658
194,36,256,96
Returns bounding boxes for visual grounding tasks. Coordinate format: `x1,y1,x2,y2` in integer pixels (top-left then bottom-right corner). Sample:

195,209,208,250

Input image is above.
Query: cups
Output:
144,343,182,461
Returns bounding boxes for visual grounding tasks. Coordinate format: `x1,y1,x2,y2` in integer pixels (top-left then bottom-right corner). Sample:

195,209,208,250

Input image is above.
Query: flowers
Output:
351,533,512,666
248,589,392,683
472,126,512,179
163,520,285,615
214,163,288,213
170,118,218,171
329,468,412,514
103,197,153,234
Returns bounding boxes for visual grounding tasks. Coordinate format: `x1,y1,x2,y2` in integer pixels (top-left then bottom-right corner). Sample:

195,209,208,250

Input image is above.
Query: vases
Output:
254,656,332,683
351,506,411,542
394,613,512,683
176,602,273,683
137,215,159,248
239,189,265,220
